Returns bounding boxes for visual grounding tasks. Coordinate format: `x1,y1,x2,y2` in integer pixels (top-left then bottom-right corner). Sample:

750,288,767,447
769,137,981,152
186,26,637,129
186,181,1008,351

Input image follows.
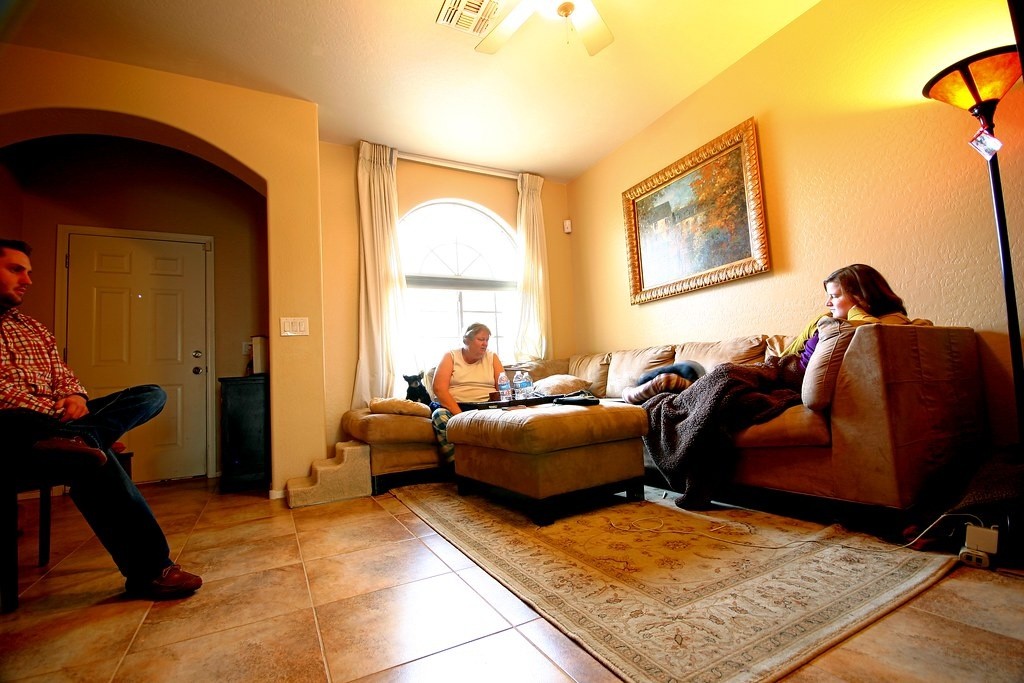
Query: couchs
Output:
342,323,988,511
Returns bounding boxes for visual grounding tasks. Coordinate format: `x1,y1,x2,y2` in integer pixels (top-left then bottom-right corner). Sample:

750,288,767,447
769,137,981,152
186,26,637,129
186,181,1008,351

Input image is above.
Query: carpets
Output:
388,481,960,683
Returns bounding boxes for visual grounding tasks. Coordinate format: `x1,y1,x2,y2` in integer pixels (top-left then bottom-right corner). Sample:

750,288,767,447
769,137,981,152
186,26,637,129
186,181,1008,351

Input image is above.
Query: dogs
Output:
403,371,432,406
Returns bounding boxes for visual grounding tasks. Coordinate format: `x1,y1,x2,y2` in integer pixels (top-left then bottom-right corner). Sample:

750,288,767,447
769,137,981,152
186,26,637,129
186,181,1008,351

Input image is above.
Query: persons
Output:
640,264,934,510
431,322,509,464
0,237,203,595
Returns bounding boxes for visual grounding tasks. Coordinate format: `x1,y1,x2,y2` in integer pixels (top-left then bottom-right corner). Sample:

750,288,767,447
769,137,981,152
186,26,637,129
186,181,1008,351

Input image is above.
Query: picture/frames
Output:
621,116,771,306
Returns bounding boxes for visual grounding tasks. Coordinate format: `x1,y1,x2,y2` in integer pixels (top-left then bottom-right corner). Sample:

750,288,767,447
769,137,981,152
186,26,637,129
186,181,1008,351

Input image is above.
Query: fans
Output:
475,0,615,57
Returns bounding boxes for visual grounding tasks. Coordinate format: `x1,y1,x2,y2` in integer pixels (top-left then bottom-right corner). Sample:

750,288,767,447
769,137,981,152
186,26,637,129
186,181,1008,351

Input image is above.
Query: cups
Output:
489,392,500,401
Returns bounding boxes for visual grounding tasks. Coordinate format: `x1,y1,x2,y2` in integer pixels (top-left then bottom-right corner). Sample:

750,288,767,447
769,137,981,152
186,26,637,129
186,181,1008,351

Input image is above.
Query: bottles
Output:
498,372,512,401
523,372,534,399
513,371,526,400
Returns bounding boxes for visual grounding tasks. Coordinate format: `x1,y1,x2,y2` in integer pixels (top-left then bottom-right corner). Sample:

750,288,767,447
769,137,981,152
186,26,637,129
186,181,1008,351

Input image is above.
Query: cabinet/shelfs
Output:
218,375,270,493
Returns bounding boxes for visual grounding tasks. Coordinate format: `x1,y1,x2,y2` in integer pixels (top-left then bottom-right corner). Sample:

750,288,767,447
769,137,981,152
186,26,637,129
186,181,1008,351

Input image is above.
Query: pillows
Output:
534,375,594,396
369,397,432,417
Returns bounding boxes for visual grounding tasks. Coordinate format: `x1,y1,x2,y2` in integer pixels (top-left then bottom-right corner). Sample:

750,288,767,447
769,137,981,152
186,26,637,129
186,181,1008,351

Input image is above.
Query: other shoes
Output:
124,563,202,595
34,435,108,470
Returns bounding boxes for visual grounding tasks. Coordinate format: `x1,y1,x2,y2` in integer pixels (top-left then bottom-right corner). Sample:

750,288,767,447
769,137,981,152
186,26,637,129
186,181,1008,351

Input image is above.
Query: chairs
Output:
0,408,133,615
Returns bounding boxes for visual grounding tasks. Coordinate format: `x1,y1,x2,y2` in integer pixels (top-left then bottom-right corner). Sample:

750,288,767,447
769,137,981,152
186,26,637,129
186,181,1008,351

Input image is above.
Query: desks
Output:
446,400,650,526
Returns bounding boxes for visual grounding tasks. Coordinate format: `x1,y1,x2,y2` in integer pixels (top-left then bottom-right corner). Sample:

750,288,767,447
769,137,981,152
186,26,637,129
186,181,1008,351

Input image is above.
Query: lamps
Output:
922,45,1024,454
